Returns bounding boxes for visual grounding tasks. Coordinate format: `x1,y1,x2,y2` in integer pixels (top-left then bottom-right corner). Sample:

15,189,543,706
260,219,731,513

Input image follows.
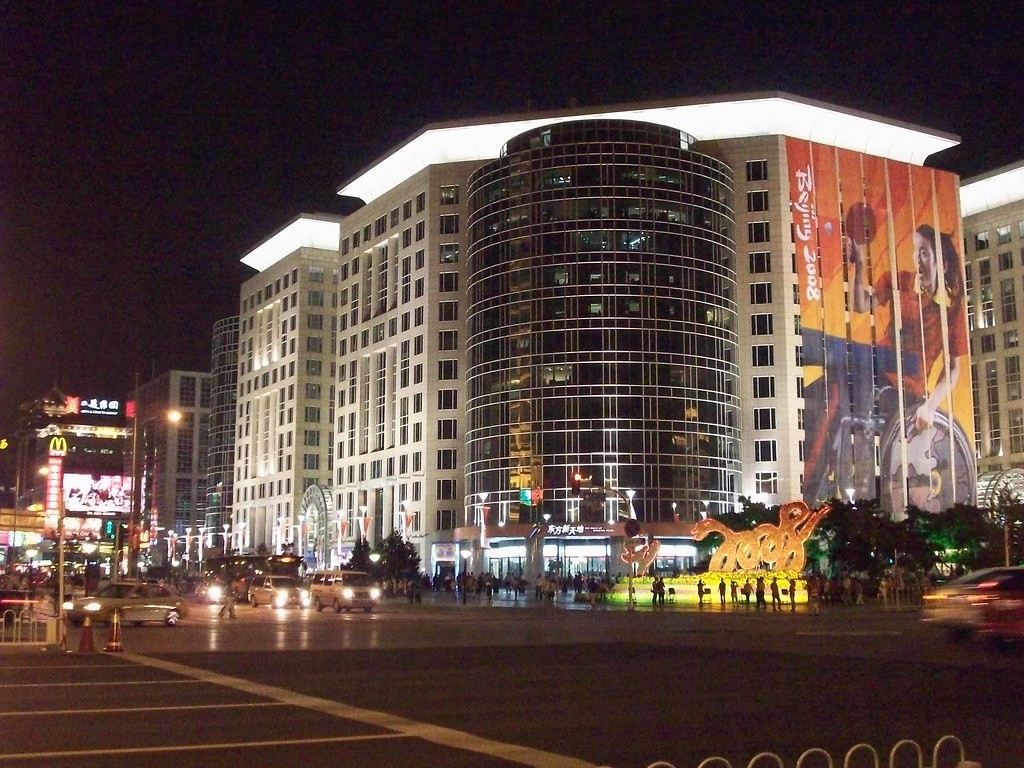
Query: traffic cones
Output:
106,607,127,652
74,611,96,654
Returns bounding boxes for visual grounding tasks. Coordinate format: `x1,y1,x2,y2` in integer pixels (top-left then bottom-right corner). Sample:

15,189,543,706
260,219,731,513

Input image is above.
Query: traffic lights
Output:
105,520,113,535
110,534,116,539
571,472,581,496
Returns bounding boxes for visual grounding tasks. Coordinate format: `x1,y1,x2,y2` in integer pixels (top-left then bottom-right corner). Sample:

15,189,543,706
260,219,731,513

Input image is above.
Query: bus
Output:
202,555,308,603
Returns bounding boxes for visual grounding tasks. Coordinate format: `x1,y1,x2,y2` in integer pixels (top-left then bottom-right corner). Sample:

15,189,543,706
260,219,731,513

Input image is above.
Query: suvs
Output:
248,575,308,609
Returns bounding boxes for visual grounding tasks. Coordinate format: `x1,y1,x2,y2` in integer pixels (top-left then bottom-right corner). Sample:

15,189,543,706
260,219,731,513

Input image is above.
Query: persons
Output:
652,573,667,603
4,572,78,626
432,571,520,602
695,576,798,612
537,570,651,603
880,572,932,612
371,574,425,606
806,576,865,615
217,579,240,621
801,223,971,500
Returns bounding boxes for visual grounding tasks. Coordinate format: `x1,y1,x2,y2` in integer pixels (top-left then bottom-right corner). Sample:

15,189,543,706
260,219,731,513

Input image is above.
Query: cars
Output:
62,580,190,629
917,565,1024,655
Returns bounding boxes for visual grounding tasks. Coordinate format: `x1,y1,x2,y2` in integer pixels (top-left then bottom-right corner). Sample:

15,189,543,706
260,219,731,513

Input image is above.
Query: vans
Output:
310,570,378,613
